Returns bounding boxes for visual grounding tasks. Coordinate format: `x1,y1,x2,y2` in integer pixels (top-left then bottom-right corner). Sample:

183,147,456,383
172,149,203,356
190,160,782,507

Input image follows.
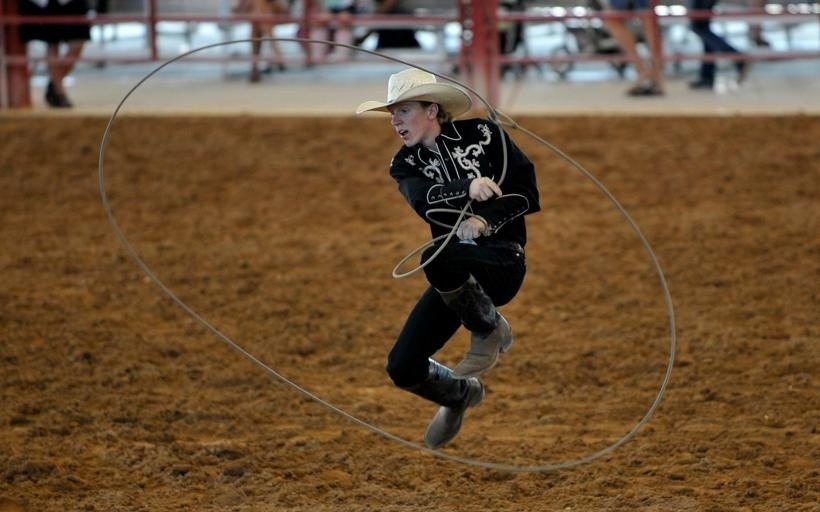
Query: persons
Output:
231,0,467,84
355,65,541,449
19,0,93,109
499,1,749,97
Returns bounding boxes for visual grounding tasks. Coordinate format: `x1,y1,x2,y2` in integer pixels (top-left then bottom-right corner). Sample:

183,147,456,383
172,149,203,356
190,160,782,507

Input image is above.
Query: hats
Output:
355,66,473,119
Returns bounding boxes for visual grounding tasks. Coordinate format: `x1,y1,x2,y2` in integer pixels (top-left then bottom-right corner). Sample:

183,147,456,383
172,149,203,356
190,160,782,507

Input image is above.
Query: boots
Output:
397,356,486,450
433,272,514,381
